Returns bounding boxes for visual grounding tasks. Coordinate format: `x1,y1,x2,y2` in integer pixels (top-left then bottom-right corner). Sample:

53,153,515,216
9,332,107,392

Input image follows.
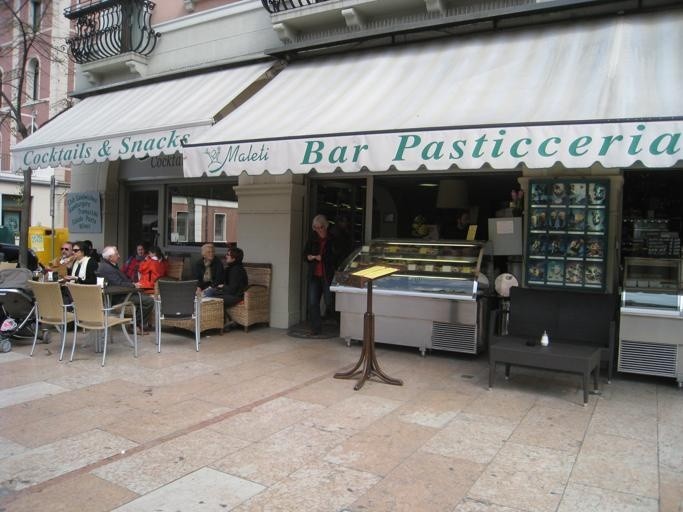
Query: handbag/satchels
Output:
61,286,73,312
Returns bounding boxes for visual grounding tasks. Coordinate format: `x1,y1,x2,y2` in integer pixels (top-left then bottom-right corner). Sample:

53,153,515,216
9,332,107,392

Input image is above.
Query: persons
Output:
455,213,468,239
49,240,168,335
212,247,248,332
193,243,225,295
303,214,338,336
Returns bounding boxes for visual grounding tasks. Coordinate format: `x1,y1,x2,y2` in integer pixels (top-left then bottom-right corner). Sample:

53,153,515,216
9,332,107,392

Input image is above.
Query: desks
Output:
117,293,225,336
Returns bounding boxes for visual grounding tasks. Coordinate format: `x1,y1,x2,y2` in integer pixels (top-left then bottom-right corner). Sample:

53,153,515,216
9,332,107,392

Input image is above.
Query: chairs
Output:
96,277,143,337
26,279,74,361
65,282,138,366
166,255,191,281
224,262,271,333
153,279,201,353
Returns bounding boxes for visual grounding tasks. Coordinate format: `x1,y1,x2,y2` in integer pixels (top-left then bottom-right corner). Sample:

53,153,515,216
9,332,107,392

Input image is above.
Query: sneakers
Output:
128,325,149,335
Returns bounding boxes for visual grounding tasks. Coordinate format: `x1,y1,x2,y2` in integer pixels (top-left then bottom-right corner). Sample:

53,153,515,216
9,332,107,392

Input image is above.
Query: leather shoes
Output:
309,328,322,335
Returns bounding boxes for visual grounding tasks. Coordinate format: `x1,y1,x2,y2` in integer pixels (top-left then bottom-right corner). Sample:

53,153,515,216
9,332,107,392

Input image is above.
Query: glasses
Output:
73,249,81,252
61,248,68,251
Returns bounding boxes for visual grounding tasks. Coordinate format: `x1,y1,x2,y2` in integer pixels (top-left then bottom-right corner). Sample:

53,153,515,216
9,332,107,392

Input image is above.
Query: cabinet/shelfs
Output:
330,242,495,356
311,181,364,248
621,254,683,307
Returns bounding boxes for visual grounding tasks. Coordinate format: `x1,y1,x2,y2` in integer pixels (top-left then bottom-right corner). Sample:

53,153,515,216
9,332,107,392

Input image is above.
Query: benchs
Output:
488,286,615,408
